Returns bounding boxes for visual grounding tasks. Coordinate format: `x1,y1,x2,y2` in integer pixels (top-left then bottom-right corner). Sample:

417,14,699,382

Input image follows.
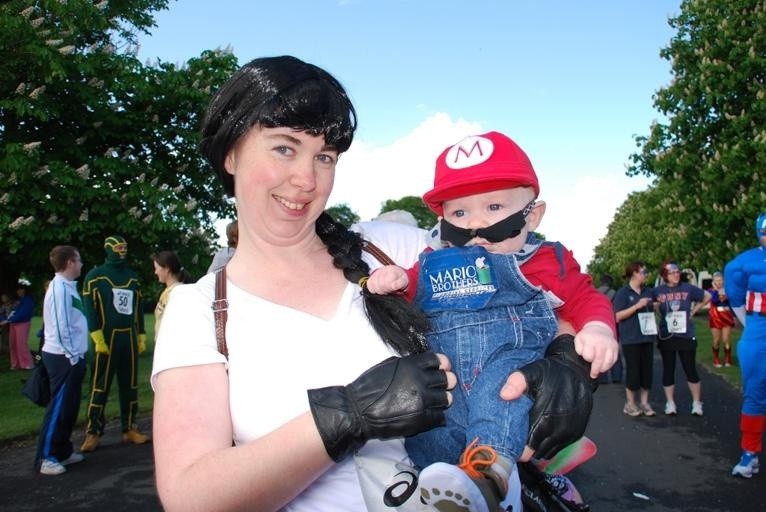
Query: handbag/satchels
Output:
21,363,51,408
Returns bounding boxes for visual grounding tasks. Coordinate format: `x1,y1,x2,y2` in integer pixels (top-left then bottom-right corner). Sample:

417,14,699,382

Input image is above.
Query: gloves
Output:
306,351,449,462
136,333,148,354
89,330,109,355
512,334,598,460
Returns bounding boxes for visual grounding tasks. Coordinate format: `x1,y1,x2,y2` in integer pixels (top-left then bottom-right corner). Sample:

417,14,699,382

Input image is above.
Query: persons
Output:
724,214,766,479
154,253,185,342
366,130,619,512
596,260,766,417
38,236,149,474
209,220,238,271
2,284,33,369
151,55,596,512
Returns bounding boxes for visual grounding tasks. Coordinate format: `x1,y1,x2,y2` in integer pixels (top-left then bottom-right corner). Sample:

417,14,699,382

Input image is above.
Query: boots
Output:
724,345,733,366
712,345,724,368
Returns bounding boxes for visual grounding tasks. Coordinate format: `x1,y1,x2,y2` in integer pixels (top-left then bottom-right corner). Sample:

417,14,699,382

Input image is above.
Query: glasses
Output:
439,196,537,248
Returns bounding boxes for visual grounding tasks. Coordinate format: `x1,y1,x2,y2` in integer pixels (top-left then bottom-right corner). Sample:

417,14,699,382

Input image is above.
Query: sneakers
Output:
665,402,679,416
691,400,704,416
59,451,84,467
417,459,504,511
122,430,149,444
622,403,641,417
38,459,66,477
80,433,98,452
732,451,760,478
639,401,656,416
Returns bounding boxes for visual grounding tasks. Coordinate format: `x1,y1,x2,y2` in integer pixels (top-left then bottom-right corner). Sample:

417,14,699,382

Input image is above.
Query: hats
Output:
422,130,540,219
